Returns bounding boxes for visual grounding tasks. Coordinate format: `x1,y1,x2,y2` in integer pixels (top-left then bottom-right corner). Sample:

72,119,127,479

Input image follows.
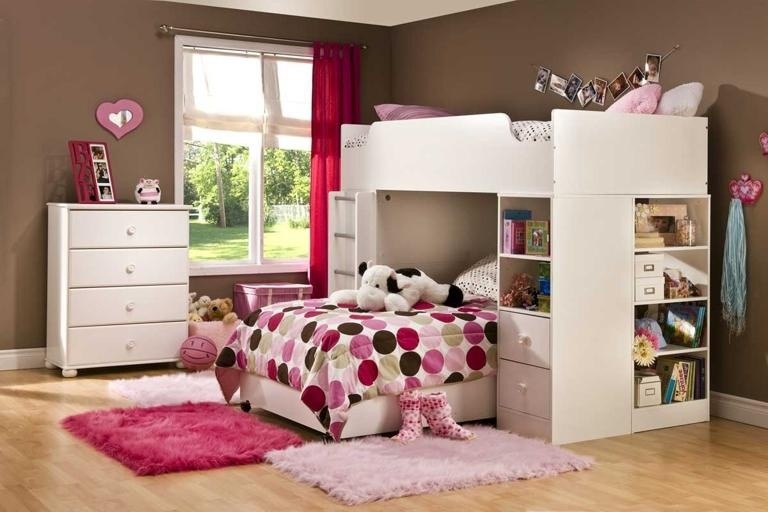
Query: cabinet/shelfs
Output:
43,198,193,380
633,196,712,434
495,196,631,448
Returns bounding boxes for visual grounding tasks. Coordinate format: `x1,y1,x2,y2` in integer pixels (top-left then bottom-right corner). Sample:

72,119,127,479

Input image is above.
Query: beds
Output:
207,249,499,441
338,111,711,195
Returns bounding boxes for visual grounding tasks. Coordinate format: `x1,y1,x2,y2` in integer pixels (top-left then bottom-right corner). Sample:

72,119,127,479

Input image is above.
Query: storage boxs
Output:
633,276,666,301
635,375,665,407
634,253,664,279
190,316,241,357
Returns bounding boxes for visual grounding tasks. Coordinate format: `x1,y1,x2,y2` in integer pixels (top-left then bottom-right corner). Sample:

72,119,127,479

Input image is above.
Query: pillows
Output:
652,80,703,116
452,253,496,301
374,102,454,119
604,81,662,113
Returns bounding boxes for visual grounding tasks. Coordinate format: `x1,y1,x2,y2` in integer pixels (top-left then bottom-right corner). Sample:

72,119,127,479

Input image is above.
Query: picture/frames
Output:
67,139,116,203
647,204,688,245
525,219,549,257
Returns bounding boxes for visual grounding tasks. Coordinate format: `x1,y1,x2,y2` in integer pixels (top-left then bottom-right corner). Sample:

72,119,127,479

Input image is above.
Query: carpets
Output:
106,366,246,407
64,404,302,477
263,420,592,505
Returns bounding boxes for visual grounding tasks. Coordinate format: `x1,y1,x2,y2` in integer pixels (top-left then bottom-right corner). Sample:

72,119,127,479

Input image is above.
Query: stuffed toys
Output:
330,262,463,314
189,292,238,325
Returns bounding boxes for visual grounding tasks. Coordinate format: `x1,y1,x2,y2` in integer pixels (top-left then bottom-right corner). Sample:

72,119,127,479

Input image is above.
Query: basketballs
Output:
181,335,216,370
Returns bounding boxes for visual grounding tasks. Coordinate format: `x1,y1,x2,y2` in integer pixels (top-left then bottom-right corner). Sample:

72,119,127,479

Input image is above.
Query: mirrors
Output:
95,99,143,139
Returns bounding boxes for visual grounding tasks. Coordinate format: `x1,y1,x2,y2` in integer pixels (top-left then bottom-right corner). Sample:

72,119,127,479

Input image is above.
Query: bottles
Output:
675,218,696,246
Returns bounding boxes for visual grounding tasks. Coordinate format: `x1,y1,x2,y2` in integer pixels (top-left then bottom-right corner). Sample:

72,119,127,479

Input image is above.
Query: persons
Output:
94,152,112,200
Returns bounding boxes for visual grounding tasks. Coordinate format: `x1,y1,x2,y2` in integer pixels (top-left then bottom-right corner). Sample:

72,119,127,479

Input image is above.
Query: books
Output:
657,305,707,404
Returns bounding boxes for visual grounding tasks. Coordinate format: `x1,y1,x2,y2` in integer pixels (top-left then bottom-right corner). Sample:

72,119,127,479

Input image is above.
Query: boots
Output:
421,391,477,440
391,390,423,442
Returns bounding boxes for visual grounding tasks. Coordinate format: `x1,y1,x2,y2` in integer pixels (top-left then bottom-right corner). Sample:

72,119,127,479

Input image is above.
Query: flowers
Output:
634,201,658,223
499,272,537,308
630,327,659,367
